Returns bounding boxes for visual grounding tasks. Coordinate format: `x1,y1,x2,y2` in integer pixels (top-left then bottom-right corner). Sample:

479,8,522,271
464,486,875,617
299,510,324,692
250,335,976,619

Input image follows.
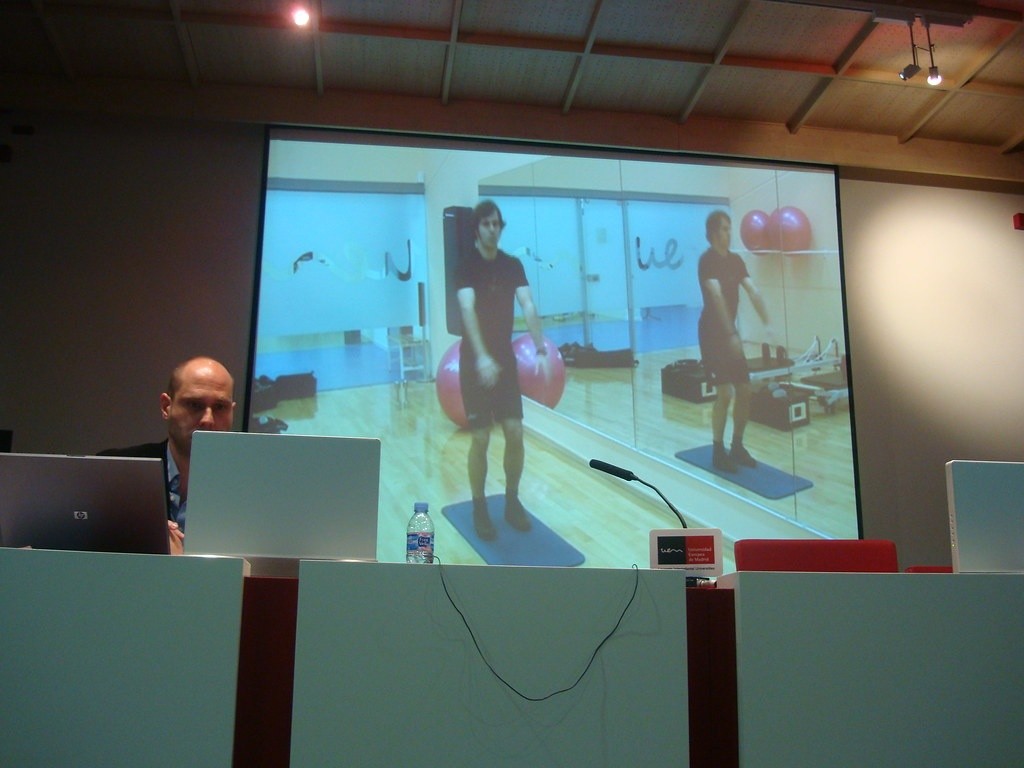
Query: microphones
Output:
589,457,687,527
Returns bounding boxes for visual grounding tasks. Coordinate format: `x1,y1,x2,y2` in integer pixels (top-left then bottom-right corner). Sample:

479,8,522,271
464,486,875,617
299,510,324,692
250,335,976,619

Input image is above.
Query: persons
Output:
697,209,776,474
454,200,548,541
96,356,236,555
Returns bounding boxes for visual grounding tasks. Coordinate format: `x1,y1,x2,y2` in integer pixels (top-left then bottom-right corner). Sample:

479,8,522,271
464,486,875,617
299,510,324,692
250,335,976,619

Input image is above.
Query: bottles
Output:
406,501,437,566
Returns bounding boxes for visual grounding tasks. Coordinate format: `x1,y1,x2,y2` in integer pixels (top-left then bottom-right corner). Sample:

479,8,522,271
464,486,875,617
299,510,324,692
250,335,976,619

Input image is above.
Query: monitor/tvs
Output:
0,452,171,556
946,459,1024,575
180,430,382,561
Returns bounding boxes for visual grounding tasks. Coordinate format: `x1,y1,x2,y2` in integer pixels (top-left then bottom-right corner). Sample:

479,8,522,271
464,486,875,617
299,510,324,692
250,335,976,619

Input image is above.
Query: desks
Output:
1,544,1023,765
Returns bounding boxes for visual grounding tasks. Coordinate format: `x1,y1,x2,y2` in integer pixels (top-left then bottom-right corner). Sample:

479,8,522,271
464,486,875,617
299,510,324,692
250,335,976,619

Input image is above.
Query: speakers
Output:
441,204,475,338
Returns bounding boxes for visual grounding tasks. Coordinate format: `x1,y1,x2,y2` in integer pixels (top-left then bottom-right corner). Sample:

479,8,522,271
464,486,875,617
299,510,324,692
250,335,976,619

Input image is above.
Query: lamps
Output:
898,63,921,81
927,66,943,86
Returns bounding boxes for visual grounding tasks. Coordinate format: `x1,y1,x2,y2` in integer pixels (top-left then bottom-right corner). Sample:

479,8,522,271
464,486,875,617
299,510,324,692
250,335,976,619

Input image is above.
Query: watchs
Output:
536,350,548,356
